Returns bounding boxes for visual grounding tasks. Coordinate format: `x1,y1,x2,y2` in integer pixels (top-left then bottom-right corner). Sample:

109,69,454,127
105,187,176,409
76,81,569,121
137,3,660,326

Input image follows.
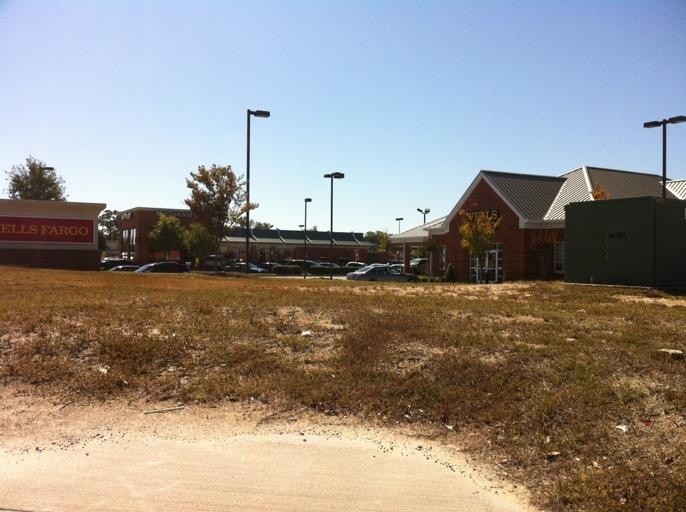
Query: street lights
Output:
304,198,311,262
396,218,404,241
325,173,343,267
418,207,429,237
643,115,686,202
246,108,269,273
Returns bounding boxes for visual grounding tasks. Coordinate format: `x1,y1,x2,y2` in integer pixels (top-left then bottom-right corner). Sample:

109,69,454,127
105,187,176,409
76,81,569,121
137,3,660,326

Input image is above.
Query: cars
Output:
347,257,427,283
223,260,345,275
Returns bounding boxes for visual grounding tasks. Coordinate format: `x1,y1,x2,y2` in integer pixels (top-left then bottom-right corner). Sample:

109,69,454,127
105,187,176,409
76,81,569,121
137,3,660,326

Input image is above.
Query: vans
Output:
102,261,190,273
